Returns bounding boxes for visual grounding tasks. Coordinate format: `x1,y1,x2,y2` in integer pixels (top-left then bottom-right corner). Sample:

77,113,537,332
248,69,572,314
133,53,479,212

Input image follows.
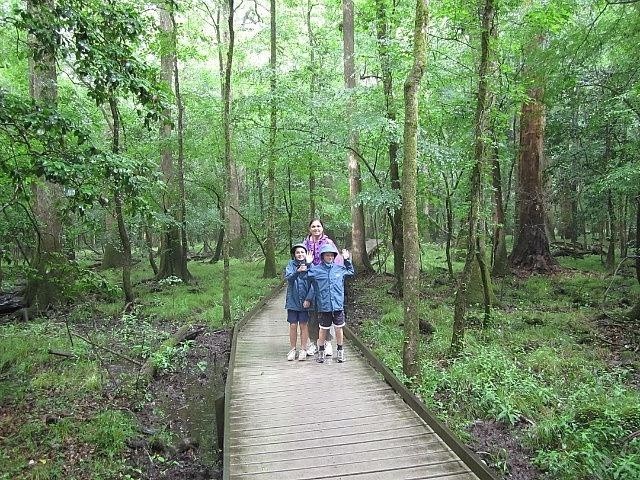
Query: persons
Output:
302,218,334,356
284,242,315,361
299,244,356,363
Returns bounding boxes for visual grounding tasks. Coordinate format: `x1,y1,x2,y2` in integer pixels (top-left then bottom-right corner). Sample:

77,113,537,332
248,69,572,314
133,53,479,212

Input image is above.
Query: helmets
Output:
319,244,338,261
290,243,308,255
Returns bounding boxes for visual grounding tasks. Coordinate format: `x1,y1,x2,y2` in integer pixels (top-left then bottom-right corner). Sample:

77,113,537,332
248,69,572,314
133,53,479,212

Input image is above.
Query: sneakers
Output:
336,348,345,363
324,343,333,355
316,350,326,363
298,350,307,361
307,344,318,356
287,349,298,361
